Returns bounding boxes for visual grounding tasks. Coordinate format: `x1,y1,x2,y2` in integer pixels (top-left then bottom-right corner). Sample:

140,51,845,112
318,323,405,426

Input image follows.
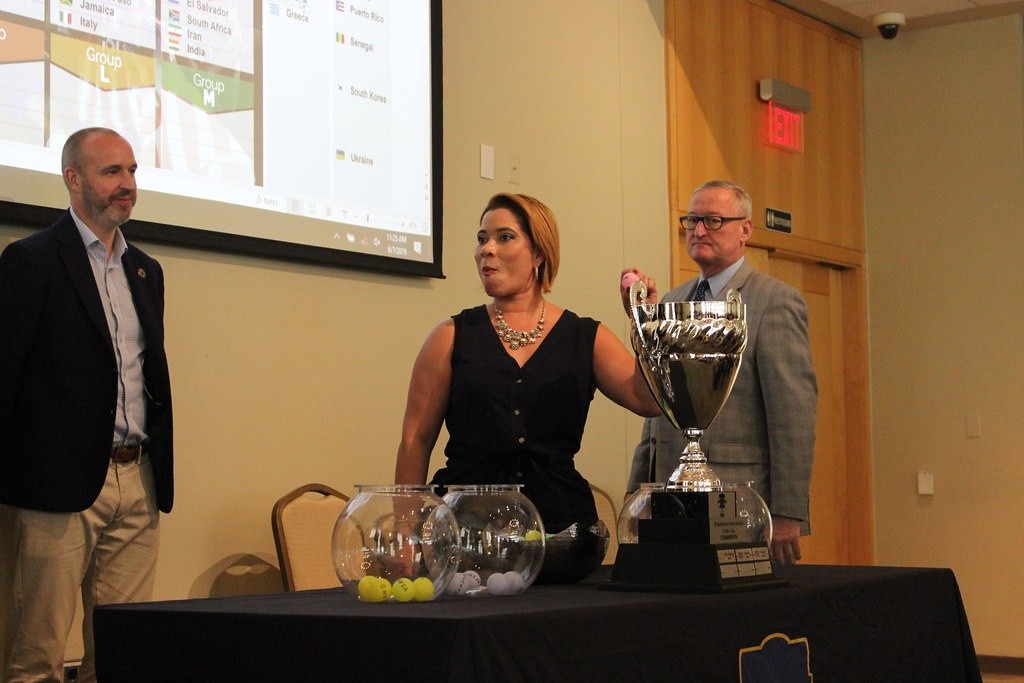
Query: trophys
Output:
596,280,790,594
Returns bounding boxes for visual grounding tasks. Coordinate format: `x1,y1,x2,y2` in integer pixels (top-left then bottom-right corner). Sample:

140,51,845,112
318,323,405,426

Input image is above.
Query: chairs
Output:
271,482,367,593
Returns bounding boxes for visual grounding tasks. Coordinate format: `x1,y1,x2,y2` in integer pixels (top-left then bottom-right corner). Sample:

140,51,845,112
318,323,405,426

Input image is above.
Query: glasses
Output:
679,215,746,231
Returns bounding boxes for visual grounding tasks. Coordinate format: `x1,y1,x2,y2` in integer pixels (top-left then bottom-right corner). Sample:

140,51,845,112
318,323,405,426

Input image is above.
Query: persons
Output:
623,180,818,566
0,127,174,683
392,193,664,578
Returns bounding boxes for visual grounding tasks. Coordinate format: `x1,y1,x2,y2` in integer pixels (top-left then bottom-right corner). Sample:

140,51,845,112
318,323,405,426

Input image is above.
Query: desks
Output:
93,563,984,683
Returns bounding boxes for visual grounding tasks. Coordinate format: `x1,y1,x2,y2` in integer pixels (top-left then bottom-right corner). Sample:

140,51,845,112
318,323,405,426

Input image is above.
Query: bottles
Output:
421,485,546,595
332,482,457,605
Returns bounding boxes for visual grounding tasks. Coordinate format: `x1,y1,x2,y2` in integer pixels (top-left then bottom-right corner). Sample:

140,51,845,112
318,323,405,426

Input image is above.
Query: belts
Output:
110,441,151,464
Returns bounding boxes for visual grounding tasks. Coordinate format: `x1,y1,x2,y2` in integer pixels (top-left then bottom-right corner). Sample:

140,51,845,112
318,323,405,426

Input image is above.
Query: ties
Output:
693,280,710,301
